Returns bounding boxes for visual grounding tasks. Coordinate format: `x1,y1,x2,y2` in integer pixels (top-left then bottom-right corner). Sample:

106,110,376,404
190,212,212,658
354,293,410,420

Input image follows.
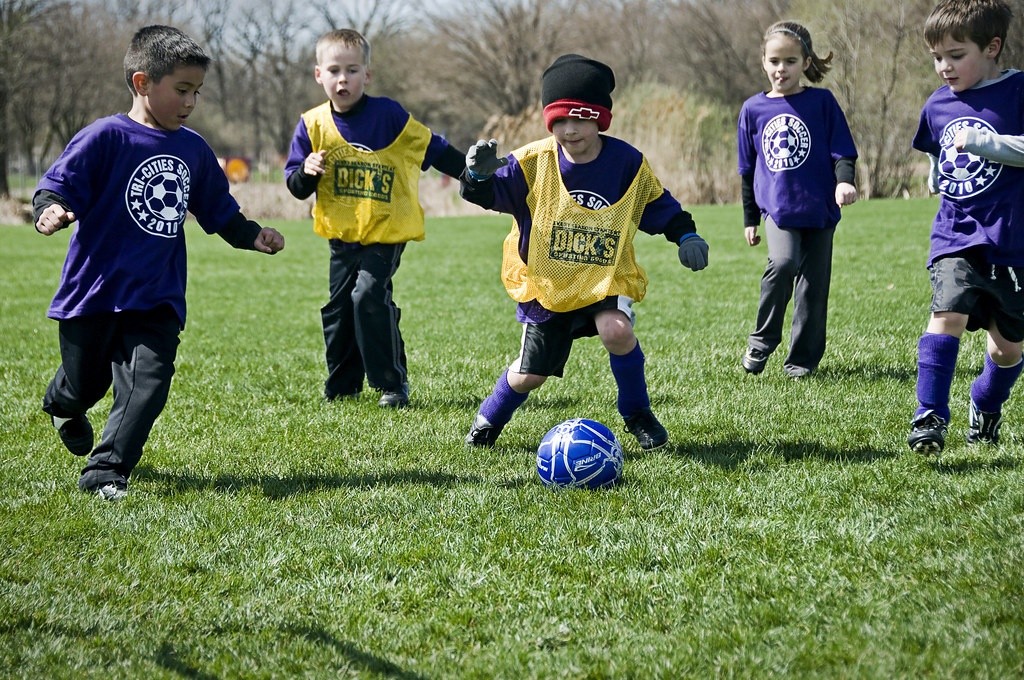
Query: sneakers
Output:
742,346,770,376
51,414,94,456
465,416,502,450
375,382,409,408
783,364,811,378
621,406,668,450
968,396,1002,446
906,409,949,456
85,481,128,501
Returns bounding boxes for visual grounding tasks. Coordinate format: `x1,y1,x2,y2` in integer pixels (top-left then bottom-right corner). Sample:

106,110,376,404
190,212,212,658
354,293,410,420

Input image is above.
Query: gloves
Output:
465,139,508,179
678,237,709,271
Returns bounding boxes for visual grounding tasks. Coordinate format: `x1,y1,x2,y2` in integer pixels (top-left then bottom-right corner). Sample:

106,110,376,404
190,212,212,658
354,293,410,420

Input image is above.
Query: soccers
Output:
533,417,626,489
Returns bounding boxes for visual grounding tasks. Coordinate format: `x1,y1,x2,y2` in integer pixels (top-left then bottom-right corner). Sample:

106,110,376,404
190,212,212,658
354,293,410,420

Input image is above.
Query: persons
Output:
280,28,472,407
907,2,1023,455
738,21,861,381
33,26,285,501
459,55,712,452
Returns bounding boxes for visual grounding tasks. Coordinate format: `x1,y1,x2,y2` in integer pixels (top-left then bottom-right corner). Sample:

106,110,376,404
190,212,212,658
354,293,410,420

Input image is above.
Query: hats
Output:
541,53,616,132
